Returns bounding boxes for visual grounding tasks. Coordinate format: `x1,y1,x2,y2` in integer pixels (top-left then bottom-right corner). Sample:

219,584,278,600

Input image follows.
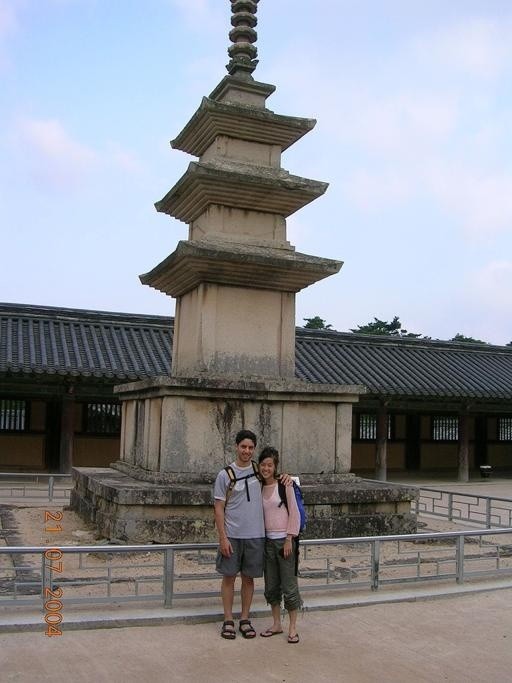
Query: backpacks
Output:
278,479,305,533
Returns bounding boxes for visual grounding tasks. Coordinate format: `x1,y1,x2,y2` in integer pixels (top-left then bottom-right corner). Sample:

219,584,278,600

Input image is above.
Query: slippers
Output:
260,628,299,643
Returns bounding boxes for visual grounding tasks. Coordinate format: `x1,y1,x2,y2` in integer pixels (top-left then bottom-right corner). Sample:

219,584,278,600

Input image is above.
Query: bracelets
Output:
286,538,292,542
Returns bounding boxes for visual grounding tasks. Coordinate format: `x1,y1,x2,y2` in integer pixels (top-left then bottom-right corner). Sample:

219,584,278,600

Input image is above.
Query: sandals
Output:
221,620,256,639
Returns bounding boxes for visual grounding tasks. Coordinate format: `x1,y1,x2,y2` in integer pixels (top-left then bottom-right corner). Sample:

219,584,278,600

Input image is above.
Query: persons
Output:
212,428,294,640
258,444,303,643
